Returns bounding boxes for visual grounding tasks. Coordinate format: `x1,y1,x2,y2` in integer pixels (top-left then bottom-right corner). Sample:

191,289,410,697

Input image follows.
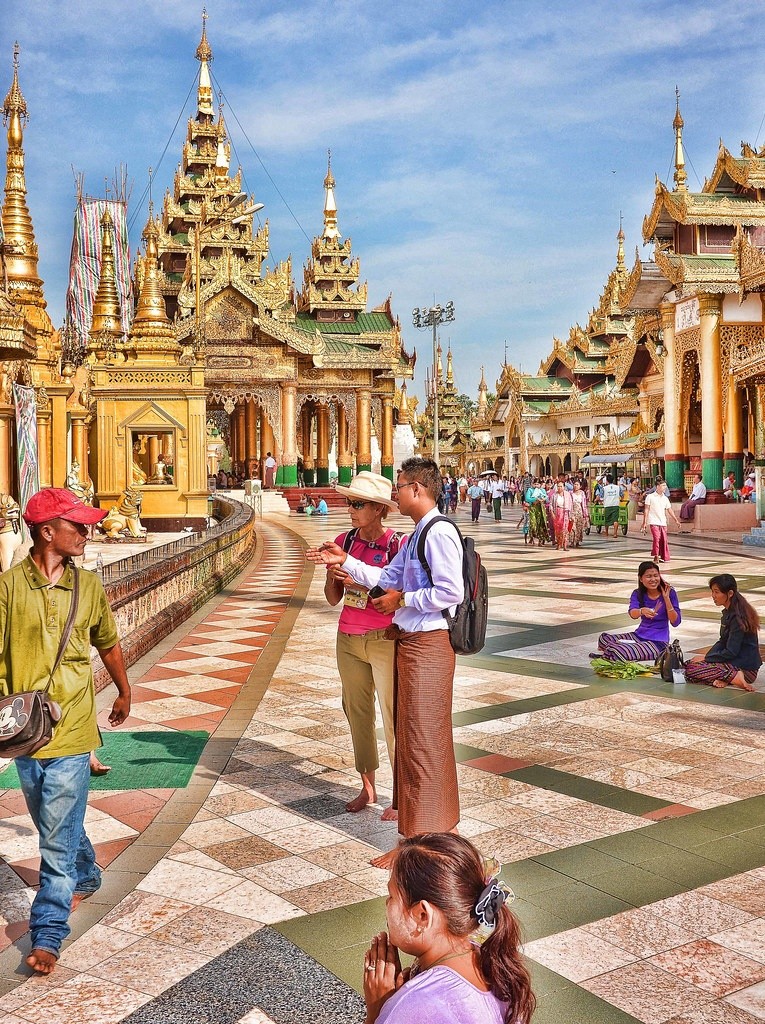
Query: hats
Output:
22,487,109,524
749,473,755,478
335,471,398,513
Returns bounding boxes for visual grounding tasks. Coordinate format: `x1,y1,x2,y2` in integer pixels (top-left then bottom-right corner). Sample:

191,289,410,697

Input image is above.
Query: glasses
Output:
348,499,372,509
397,481,428,492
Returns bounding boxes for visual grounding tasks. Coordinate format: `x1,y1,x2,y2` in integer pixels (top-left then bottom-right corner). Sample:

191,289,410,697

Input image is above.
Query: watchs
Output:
399,592,406,607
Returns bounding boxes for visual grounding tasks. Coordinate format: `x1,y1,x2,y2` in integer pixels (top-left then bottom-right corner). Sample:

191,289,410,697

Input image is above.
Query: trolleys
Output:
581,453,633,535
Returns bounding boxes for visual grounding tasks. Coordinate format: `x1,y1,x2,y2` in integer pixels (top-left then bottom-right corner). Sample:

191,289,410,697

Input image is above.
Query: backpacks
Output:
407,516,488,655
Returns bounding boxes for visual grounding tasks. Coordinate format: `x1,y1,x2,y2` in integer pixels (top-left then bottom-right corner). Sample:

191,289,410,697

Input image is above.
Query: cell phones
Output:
370,585,387,598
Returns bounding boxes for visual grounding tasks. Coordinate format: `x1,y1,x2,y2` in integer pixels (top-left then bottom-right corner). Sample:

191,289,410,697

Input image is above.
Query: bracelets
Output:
668,606,674,612
640,608,643,614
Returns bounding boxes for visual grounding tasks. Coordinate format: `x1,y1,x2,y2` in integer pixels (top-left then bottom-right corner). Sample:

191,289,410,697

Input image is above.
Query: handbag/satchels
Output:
487,505,492,513
655,639,687,681
0,690,62,758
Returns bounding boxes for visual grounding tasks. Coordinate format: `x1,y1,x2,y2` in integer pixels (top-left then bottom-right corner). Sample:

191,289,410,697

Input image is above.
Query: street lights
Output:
412,300,456,465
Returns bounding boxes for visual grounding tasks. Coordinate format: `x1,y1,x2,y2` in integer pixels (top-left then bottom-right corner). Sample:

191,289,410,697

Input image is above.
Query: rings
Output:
367,966,375,971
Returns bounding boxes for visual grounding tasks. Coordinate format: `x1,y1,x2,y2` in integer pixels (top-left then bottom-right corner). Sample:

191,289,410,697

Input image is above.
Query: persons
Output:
0,487,131,972
598,561,681,664
641,481,681,564
297,493,328,515
723,471,756,502
363,831,537,1024
305,458,465,870
90,749,111,774
149,454,169,479
685,574,763,692
679,474,706,523
436,470,671,551
264,452,275,488
67,461,85,491
212,469,247,489
324,470,409,821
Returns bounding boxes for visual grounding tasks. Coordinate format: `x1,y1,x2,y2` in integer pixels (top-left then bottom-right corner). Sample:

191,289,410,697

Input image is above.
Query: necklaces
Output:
414,938,475,971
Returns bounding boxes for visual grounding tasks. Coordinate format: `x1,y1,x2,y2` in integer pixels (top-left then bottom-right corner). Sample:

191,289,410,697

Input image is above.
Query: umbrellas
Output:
480,469,498,478
